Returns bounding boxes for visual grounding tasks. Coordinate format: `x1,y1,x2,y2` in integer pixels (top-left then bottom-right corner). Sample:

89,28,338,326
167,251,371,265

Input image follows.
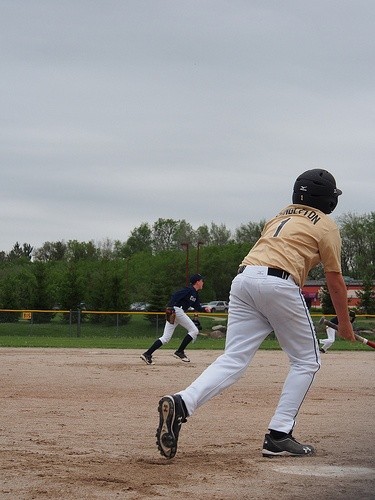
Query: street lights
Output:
196,241,205,274
181,242,189,287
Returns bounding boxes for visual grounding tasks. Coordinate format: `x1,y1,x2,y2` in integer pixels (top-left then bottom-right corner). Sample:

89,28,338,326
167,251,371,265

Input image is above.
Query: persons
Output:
318,306,356,353
140,273,210,365
157,169,356,459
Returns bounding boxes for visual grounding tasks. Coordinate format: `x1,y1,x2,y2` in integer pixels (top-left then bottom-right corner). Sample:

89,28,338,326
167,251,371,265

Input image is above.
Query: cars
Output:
200,302,208,309
129,301,197,312
201,301,229,313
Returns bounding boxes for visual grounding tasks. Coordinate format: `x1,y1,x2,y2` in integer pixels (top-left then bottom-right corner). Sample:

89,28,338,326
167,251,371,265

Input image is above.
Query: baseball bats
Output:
319,316,375,349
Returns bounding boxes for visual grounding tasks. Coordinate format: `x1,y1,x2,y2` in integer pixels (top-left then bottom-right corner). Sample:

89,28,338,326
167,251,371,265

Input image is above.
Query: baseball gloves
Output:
165,308,176,324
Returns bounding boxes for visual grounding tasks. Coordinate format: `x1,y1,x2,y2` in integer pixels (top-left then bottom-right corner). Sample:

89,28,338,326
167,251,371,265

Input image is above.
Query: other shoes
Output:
319,347,326,353
173,350,190,362
317,338,319,344
140,352,153,365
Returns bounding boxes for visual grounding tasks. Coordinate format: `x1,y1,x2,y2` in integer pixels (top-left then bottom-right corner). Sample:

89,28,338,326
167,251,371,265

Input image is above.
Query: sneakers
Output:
155,394,188,459
262,430,314,457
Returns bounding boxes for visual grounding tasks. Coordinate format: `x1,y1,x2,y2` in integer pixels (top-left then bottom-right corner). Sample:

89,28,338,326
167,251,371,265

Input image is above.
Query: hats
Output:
190,273,207,284
349,310,357,318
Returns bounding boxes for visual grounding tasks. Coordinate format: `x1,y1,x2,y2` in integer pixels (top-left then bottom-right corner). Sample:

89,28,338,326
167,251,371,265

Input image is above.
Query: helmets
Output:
292,168,344,215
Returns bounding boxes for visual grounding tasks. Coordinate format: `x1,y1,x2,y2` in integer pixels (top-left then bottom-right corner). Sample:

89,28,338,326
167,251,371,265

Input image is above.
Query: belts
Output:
237,266,290,280
174,304,184,309
